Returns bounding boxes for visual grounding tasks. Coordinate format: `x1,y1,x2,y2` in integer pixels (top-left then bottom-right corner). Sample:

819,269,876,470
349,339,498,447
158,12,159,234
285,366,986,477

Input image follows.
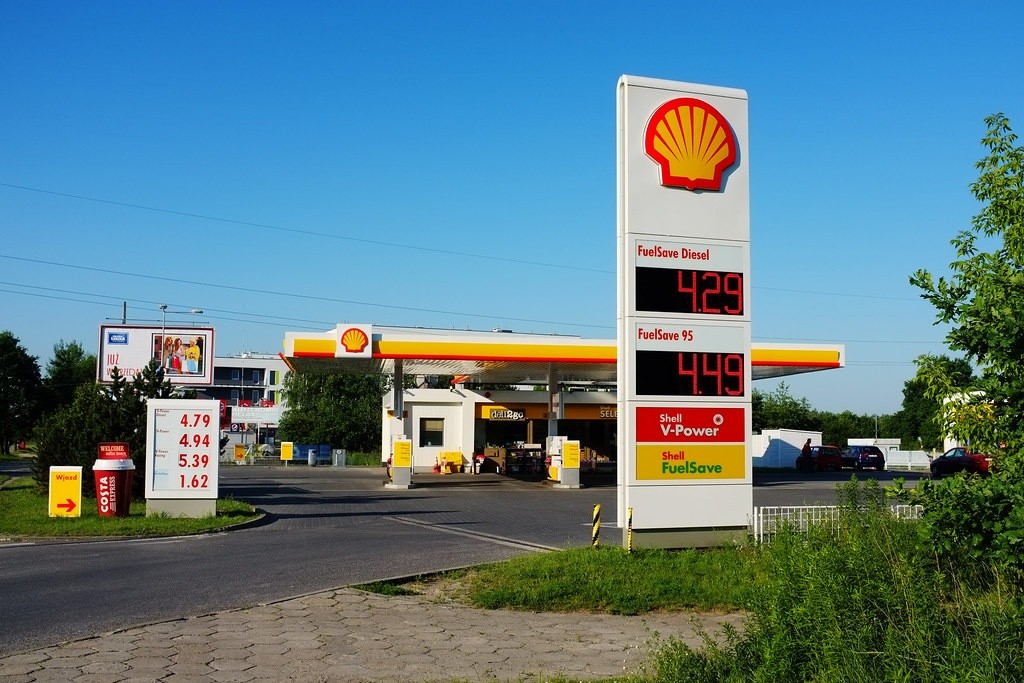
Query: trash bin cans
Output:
308,449,317,467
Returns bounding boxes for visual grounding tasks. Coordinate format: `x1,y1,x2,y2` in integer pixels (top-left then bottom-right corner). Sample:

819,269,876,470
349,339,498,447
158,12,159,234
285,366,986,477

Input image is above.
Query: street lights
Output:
158,304,204,399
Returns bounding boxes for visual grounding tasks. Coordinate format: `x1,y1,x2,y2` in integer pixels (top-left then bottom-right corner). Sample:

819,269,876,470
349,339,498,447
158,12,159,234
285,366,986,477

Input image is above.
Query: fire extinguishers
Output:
545,457,551,472
386,457,392,478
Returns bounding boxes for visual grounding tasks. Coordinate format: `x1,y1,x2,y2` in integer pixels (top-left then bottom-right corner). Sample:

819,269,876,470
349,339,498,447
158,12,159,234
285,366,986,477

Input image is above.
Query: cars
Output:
795,445,841,470
841,445,886,472
930,448,999,478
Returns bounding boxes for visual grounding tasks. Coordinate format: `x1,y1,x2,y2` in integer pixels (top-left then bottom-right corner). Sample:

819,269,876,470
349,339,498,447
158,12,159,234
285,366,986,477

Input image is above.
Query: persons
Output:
159,337,203,374
802,438,813,472
